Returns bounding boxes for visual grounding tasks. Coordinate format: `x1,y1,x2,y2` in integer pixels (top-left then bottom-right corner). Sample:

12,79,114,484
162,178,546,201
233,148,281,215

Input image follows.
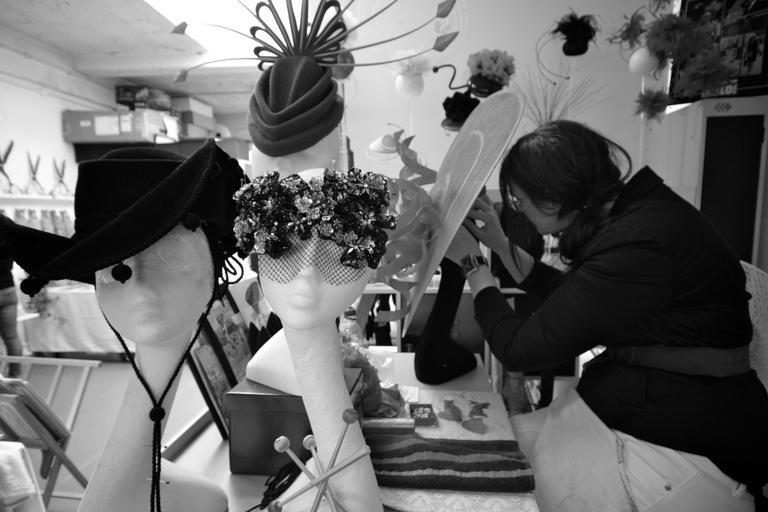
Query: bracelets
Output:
459,251,488,275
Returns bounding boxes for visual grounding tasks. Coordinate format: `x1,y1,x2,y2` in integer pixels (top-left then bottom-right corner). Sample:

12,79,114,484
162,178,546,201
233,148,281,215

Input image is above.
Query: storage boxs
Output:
223,367,363,475
62,85,214,145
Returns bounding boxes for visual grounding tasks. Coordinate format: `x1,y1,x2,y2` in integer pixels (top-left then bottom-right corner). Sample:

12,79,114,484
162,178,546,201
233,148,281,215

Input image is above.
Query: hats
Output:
0,137,254,299
247,55,345,158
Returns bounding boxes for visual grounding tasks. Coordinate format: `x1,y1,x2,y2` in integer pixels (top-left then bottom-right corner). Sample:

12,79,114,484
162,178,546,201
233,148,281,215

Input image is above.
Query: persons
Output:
440,118,767,511
229,155,400,512
493,205,577,379
1,134,256,512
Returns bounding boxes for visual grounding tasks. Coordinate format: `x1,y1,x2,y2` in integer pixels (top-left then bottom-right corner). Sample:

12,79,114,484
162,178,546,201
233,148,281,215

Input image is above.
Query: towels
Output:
365,434,536,493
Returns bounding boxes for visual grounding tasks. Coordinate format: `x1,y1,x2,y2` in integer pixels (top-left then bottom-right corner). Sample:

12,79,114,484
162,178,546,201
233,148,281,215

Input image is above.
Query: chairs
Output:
738,259,768,391
